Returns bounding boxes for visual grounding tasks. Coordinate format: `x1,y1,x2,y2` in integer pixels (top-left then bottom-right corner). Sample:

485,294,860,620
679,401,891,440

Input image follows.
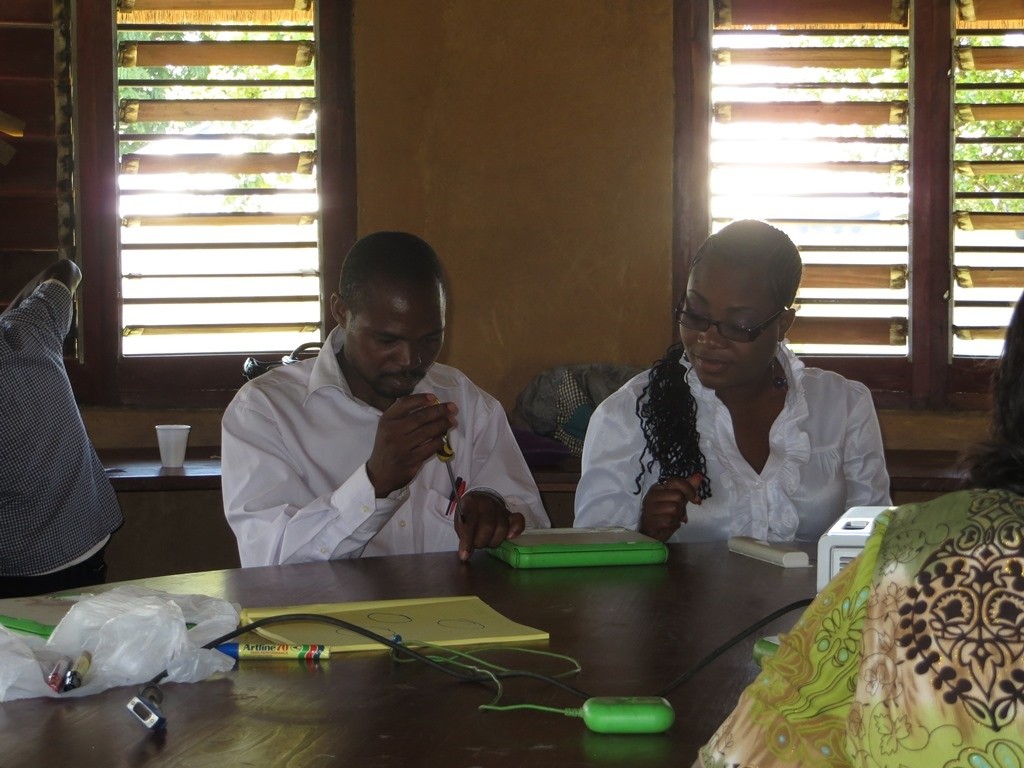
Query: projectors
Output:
817,505,905,596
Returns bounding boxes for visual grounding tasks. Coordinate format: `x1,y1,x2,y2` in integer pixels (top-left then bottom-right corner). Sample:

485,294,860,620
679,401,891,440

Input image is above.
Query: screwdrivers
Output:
426,398,465,522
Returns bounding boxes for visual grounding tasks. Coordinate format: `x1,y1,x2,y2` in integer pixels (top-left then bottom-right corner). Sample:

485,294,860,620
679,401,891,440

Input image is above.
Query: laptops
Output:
487,526,670,568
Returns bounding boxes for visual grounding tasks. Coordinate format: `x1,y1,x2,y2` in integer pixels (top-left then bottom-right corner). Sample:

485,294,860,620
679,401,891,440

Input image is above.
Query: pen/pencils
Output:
445,477,467,516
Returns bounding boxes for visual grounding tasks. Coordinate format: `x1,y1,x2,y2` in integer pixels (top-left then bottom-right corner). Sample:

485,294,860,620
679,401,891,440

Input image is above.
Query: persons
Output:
574,219,892,542
0,260,124,600
691,293,1024,768
220,230,552,570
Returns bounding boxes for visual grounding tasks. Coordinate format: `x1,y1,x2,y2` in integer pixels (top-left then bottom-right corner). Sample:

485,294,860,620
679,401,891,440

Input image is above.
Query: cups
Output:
154,424,191,468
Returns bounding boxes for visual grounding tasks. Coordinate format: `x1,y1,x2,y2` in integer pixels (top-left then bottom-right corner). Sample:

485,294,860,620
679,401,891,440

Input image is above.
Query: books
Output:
244,596,549,652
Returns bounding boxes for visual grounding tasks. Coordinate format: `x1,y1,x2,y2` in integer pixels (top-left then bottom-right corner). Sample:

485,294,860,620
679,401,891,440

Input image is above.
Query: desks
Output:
512,428,583,493
94,445,222,492
0,537,818,768
884,448,964,493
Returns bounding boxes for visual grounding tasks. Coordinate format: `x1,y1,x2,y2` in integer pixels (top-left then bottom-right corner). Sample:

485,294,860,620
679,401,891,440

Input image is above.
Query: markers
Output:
218,642,331,661
64,650,90,692
49,655,69,692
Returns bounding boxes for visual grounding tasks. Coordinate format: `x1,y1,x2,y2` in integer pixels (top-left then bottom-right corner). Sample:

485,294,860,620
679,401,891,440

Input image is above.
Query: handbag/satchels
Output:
0,585,240,704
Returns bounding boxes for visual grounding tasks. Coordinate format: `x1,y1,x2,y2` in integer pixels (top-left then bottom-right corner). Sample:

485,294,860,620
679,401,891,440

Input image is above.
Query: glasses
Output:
673,290,789,343
